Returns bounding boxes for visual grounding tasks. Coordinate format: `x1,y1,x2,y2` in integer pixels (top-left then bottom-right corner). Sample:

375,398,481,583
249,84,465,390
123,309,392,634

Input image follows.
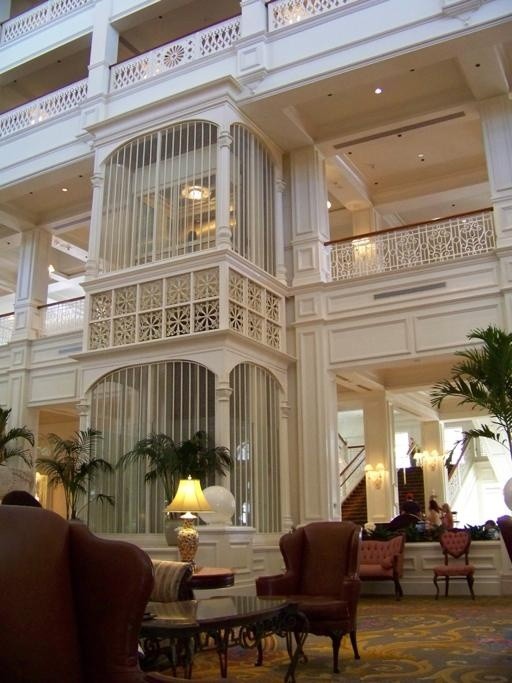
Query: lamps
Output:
174,179,214,209
162,472,216,573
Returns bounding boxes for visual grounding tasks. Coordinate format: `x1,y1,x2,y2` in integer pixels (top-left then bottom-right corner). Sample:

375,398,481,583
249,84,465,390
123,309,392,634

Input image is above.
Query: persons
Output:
407,436,418,467
424,499,443,529
479,519,499,540
399,491,421,517
439,502,453,529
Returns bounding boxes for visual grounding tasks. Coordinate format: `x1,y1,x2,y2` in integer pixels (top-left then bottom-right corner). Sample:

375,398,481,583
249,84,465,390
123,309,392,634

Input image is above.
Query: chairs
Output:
255,520,362,673
431,529,476,601
362,531,405,601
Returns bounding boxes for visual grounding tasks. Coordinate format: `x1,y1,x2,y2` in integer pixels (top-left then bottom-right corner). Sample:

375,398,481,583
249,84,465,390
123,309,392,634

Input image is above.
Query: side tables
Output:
190,566,234,654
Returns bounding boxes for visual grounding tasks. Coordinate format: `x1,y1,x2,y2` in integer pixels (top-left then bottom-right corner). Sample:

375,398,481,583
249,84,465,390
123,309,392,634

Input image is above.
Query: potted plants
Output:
113,430,235,546
